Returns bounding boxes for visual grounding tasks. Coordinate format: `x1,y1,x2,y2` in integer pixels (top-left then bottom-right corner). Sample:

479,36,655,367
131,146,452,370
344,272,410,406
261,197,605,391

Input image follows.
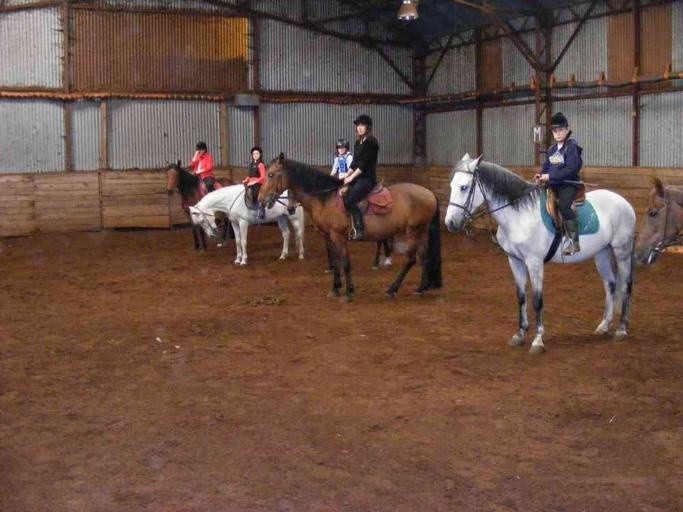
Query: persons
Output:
330,139,353,179
242,146,267,220
189,142,215,191
344,115,379,240
532,112,584,254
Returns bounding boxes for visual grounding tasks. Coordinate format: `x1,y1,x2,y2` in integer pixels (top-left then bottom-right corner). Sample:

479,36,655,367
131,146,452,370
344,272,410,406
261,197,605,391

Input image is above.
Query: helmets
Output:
549,112,568,137
353,114,373,131
336,138,349,150
195,141,206,150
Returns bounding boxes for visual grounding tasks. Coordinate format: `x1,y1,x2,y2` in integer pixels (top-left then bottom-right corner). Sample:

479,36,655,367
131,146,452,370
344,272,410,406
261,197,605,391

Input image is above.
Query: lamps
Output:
396,0,418,21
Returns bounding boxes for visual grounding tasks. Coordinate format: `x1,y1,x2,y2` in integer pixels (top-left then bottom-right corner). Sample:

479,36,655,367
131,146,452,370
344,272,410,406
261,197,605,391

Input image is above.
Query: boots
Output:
346,206,364,241
562,217,580,252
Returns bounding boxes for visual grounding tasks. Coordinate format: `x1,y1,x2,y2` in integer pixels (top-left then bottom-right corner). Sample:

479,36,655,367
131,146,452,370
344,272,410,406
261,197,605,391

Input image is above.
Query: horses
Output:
633,176,683,267
188,183,306,266
443,152,637,355
256,151,442,306
165,159,237,254
